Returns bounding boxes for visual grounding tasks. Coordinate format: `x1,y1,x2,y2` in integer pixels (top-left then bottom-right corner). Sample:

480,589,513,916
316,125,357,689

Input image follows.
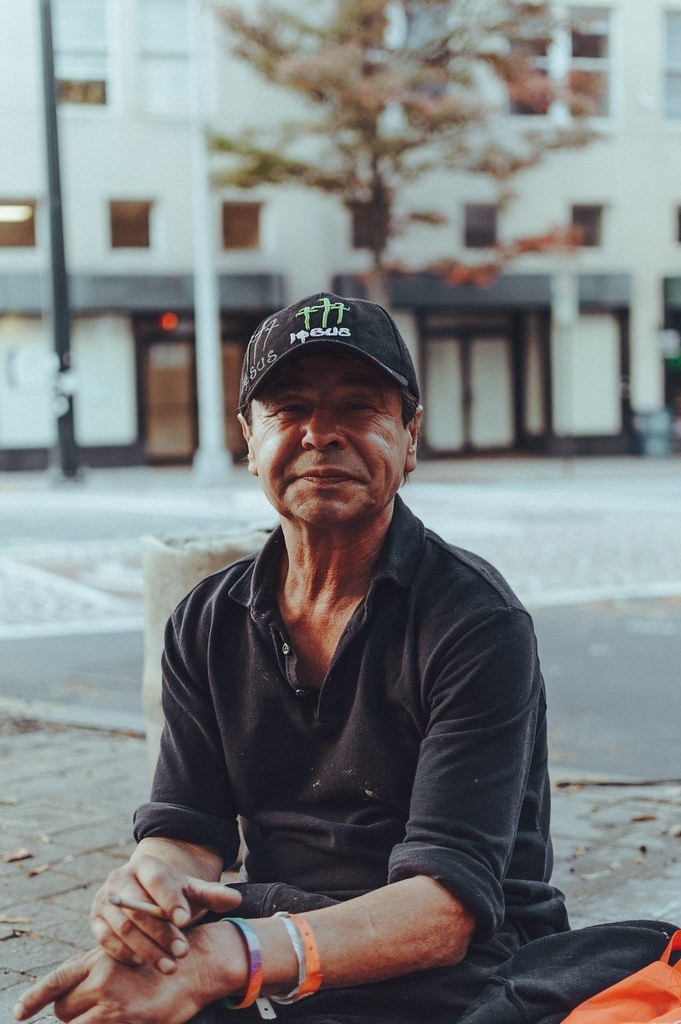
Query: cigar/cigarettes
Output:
108,896,168,921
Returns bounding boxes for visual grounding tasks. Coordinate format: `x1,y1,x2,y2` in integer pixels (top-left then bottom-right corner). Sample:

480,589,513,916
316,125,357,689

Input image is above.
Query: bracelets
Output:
220,910,324,1020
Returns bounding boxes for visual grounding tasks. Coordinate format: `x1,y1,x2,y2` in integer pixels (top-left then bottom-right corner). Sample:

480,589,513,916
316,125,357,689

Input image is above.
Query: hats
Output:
239,292,421,416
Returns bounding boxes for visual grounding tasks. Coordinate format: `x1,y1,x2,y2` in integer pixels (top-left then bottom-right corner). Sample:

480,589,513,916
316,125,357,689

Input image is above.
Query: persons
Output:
13,291,573,1024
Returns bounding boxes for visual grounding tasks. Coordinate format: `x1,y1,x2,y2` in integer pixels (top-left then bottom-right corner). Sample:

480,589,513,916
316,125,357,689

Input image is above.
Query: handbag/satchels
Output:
559,930,681,1024
